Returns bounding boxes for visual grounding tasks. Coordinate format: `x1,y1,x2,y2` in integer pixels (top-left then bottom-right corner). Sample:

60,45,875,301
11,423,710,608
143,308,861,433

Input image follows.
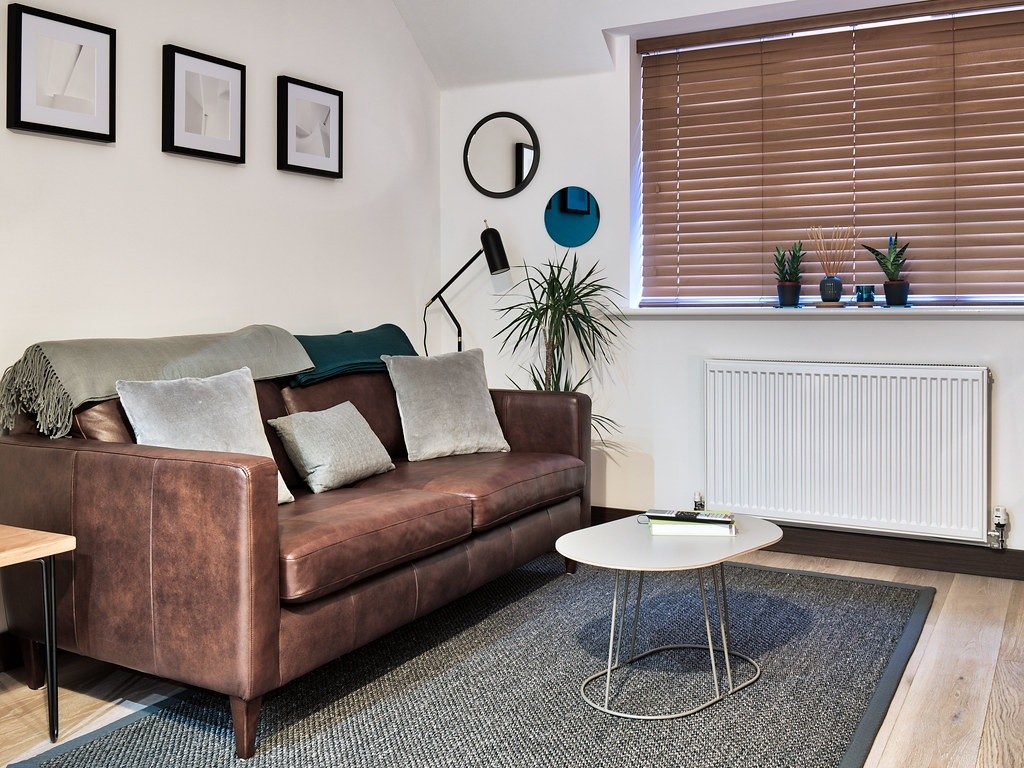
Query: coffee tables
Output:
556,512,783,720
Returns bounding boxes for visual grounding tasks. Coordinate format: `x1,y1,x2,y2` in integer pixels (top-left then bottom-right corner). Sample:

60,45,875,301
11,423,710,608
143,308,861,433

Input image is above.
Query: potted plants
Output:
493,243,631,449
774,240,806,306
861,232,909,305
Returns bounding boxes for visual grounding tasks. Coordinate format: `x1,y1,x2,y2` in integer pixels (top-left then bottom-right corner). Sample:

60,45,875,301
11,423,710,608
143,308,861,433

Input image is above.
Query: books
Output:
649,517,738,538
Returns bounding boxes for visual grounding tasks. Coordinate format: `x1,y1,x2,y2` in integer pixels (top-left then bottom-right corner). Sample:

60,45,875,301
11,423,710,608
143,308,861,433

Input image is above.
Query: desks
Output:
0,525,76,744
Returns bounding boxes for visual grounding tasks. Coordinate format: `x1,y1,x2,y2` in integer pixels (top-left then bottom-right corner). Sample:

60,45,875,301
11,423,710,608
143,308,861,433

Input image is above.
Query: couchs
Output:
0,324,592,759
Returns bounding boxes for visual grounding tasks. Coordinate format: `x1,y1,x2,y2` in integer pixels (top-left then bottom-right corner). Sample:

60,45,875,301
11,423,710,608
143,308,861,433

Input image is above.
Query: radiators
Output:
703,358,988,543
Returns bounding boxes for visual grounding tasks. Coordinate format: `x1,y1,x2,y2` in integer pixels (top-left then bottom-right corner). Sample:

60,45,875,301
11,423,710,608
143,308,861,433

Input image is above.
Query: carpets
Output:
6,550,935,768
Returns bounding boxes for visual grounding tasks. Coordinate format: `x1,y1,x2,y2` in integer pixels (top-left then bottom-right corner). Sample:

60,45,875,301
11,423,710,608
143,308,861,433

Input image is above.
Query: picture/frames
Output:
162,44,247,165
7,3,116,143
559,187,590,215
515,142,534,187
277,75,343,178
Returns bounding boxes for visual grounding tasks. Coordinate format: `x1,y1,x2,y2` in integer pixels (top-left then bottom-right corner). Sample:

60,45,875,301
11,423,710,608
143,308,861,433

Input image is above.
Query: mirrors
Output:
463,111,540,199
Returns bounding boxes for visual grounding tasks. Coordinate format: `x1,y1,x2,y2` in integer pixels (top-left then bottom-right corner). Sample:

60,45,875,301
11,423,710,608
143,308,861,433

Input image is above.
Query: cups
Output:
856,286,875,308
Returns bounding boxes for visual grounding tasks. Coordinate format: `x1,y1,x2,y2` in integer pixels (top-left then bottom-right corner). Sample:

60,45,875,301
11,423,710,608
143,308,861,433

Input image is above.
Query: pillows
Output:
113,366,296,505
381,349,511,460
268,399,395,494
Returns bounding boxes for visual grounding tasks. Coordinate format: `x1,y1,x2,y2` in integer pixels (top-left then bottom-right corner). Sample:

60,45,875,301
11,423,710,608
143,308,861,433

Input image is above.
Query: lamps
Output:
426,221,510,355
